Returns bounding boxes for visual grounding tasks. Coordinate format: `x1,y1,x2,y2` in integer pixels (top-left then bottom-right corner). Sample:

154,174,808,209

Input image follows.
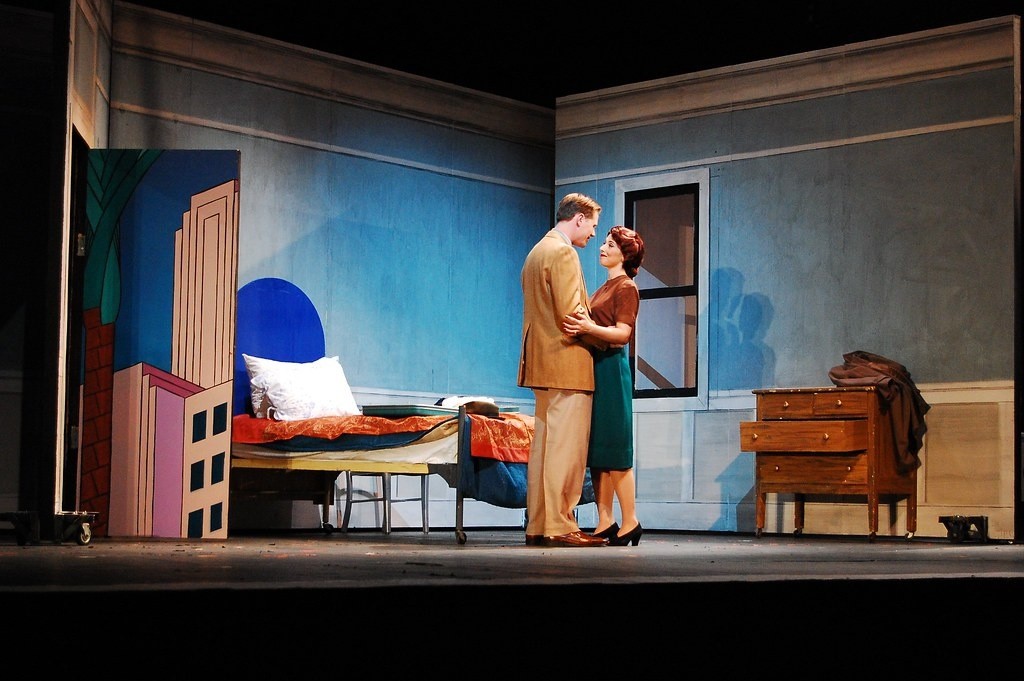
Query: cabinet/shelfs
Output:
740,386,917,542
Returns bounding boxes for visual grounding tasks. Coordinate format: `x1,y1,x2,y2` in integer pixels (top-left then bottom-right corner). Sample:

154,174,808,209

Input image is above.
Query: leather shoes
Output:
525,535,545,546
544,530,608,547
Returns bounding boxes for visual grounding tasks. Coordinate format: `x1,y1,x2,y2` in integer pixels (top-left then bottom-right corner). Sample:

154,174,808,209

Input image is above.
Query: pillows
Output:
242,354,362,420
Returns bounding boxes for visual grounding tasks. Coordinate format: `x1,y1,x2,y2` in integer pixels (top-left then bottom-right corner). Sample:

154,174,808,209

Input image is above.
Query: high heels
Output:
605,523,643,546
589,522,620,540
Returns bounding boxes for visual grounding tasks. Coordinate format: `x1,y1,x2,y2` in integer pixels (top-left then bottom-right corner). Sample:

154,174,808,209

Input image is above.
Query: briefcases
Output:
362,406,520,415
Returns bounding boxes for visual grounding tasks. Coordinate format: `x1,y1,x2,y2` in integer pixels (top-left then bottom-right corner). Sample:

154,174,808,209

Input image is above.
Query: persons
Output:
563,226,644,547
518,193,624,547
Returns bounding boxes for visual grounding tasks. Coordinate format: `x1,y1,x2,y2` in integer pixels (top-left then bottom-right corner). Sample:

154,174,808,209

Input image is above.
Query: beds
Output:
231,277,596,544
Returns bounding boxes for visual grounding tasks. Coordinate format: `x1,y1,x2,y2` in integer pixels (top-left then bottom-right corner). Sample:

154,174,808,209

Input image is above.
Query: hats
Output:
463,401,506,420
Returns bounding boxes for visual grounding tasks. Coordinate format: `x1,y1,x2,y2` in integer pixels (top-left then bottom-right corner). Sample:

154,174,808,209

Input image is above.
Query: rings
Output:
575,330,578,334
